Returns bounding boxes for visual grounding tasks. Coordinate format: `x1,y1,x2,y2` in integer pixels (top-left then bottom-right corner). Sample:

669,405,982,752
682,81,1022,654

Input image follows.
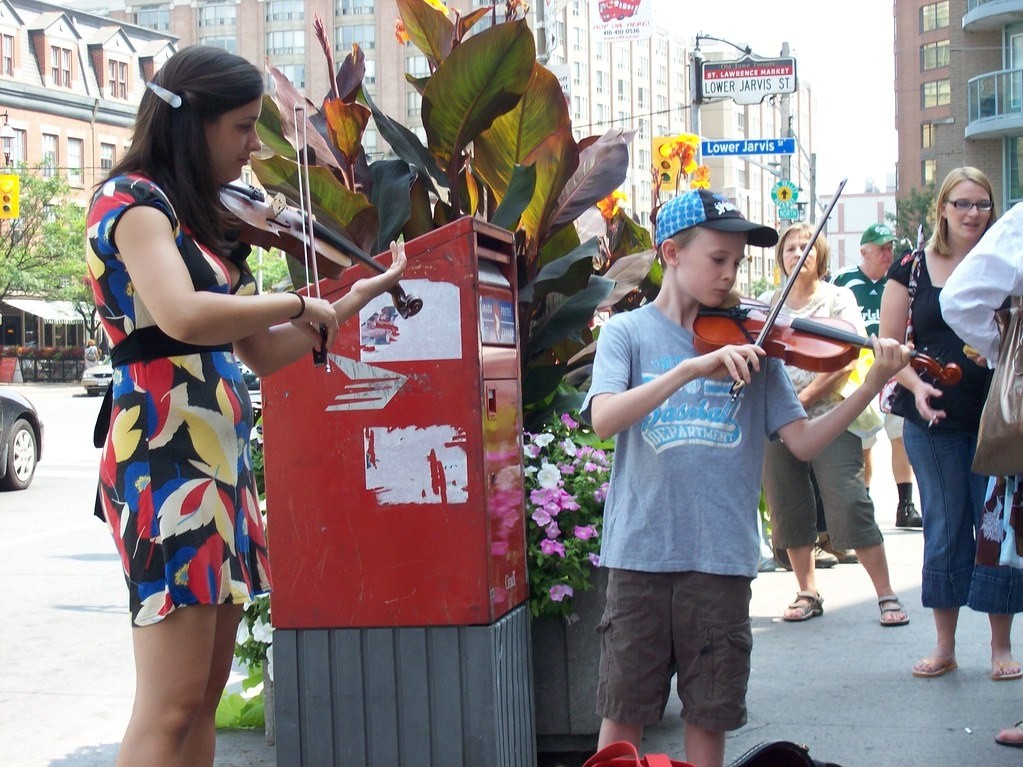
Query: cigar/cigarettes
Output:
927,412,938,427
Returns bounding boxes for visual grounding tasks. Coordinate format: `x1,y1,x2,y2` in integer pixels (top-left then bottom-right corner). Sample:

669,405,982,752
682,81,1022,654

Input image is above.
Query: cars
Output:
0,386,44,491
82,356,113,398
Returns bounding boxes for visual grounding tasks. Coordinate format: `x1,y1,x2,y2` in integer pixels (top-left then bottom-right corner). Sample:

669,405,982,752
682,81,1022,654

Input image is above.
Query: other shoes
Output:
813,534,858,567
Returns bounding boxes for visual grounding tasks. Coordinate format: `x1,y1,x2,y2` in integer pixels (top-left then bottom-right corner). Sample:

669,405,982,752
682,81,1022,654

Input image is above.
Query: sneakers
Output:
894,501,923,531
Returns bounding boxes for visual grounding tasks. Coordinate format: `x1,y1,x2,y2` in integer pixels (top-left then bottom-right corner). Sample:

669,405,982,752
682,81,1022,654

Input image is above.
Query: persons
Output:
84,340,102,370
82,47,409,767
828,223,923,530
586,190,914,767
770,459,860,569
755,221,910,627
870,166,1023,683
937,198,1023,746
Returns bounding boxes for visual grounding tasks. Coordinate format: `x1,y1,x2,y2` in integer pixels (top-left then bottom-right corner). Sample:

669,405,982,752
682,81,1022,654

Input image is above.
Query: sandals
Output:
876,595,906,625
784,591,826,624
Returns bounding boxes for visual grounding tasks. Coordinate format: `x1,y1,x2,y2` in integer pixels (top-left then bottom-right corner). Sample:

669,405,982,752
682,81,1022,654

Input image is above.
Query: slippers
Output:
995,720,1023,746
914,656,960,679
987,659,1021,680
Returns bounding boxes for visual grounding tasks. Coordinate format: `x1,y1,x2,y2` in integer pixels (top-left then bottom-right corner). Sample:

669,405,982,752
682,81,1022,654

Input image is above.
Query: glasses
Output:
944,196,996,214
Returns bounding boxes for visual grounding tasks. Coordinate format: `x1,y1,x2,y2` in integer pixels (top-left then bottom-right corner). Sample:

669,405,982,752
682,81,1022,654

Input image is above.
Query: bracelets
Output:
286,290,306,320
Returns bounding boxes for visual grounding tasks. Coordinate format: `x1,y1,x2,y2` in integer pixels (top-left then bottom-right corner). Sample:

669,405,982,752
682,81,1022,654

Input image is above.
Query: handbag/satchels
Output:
969,303,1023,477
86,349,95,362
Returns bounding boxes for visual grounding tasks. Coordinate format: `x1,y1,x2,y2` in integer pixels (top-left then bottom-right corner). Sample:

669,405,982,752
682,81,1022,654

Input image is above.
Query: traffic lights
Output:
1,174,19,219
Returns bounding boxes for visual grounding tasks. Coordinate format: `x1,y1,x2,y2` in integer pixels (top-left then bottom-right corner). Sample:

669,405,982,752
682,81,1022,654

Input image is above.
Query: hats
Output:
656,188,779,248
860,223,901,246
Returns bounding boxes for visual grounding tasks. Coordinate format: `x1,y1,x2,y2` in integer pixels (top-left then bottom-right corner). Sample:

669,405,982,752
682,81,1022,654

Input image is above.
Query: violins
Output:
214,179,424,319
692,296,964,387
